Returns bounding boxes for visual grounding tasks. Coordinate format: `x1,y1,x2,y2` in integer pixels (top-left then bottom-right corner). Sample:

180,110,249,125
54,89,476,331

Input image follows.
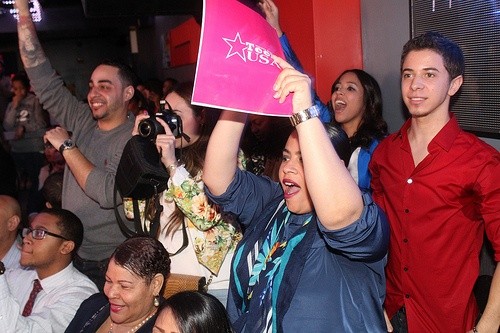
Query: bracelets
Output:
166,161,180,174
289,104,320,127
0,261,6,275
473,325,479,333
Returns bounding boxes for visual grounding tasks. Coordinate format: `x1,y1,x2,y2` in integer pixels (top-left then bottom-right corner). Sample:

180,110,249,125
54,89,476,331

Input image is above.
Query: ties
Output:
22,279,43,317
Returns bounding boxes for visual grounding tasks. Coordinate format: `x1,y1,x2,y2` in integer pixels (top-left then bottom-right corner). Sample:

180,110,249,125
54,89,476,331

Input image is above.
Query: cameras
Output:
138,100,183,139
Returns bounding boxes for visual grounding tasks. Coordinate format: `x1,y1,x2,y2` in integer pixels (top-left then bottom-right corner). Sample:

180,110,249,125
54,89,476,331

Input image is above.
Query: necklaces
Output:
108,312,155,333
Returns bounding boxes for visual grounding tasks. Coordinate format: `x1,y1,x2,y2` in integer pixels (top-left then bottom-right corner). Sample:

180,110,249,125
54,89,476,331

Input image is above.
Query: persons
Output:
12,1,136,293
368,32,500,333
0,208,100,332
254,0,389,196
0,70,180,208
65,236,171,333
152,290,231,333
241,113,294,183
0,195,36,271
124,85,246,290
200,54,391,333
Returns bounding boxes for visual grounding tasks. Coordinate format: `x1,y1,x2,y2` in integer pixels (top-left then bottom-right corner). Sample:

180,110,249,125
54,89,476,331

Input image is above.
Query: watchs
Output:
59,139,78,154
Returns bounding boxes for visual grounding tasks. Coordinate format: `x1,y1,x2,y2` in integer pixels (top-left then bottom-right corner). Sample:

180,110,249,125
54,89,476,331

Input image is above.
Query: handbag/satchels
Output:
113,135,170,239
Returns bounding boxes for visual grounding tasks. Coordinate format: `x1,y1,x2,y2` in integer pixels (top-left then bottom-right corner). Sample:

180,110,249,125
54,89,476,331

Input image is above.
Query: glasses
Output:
22,228,69,241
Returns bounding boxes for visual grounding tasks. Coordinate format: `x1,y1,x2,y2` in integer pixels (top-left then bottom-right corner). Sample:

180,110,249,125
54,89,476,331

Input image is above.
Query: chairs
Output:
162,274,206,299
